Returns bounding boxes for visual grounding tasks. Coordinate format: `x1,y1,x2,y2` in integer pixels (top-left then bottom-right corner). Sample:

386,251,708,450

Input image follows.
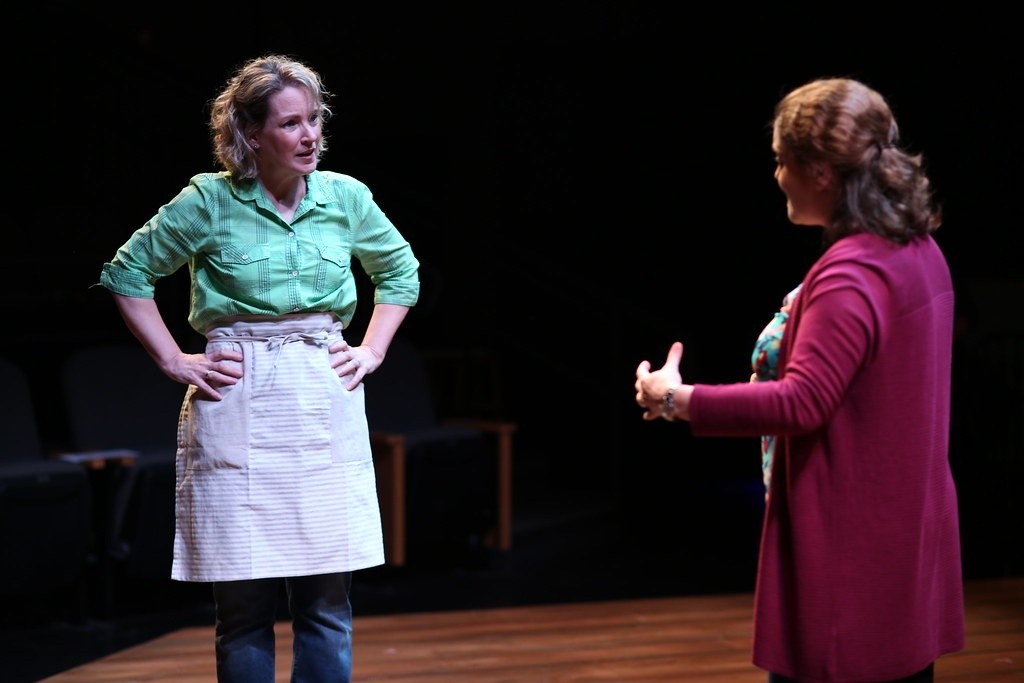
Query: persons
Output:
89,51,423,683
634,76,967,683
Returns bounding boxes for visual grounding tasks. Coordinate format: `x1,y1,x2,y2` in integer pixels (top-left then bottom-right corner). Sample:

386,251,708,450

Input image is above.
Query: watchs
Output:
658,383,682,422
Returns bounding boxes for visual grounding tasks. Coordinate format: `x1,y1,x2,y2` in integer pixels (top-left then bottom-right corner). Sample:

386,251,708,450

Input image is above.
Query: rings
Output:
205,371,210,379
